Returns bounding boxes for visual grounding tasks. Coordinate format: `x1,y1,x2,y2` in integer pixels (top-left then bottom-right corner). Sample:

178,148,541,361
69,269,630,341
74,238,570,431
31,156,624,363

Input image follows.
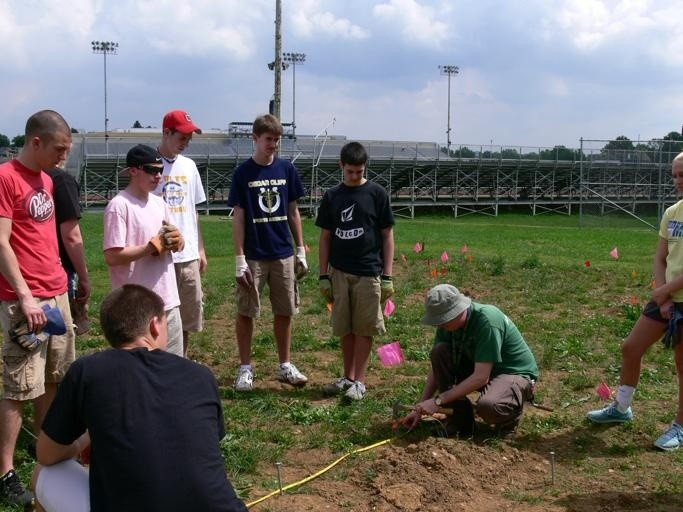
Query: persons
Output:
393,283,539,439
226,113,311,392
312,142,397,403
53,124,91,335
583,150,682,454
0,109,80,507
99,143,188,359
24,282,246,512
154,108,209,359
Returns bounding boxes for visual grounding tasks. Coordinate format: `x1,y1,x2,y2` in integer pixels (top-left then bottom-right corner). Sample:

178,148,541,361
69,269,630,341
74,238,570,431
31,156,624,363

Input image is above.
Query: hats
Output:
163,109,201,135
420,283,472,325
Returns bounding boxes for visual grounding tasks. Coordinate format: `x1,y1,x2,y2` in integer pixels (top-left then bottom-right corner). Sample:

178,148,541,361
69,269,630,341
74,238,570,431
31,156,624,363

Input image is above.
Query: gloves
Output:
235,255,253,290
319,274,334,304
295,246,309,280
162,220,185,253
7,304,66,351
149,227,170,257
380,274,395,304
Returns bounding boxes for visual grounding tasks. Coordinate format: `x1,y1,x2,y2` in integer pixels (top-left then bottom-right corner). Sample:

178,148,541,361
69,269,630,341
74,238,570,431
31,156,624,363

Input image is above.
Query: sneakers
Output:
586,401,632,423
345,379,366,400
0,469,31,505
236,369,253,391
279,364,308,385
332,376,355,391
654,423,683,452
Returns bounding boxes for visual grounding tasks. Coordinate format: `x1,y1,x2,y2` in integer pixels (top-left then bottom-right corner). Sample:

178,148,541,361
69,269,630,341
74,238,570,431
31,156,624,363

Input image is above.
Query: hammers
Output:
391,397,454,417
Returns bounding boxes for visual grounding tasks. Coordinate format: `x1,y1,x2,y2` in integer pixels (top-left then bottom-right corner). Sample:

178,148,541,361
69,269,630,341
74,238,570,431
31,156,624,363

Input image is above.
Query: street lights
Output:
282,52,306,159
91,40,119,160
438,65,460,162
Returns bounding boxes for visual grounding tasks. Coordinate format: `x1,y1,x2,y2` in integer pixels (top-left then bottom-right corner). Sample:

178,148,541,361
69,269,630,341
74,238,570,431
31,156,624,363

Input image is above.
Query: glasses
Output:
142,164,165,176
126,145,164,167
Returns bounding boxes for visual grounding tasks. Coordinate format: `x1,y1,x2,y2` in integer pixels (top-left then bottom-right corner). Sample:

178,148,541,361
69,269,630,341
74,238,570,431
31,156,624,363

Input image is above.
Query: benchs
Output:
75,159,678,199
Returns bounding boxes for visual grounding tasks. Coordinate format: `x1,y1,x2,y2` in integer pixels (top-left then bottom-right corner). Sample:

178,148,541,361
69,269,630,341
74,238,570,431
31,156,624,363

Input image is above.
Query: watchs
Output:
433,394,446,409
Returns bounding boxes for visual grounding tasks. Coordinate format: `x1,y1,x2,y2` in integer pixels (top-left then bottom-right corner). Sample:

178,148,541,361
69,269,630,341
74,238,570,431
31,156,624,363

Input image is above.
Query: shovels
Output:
527,394,555,413
